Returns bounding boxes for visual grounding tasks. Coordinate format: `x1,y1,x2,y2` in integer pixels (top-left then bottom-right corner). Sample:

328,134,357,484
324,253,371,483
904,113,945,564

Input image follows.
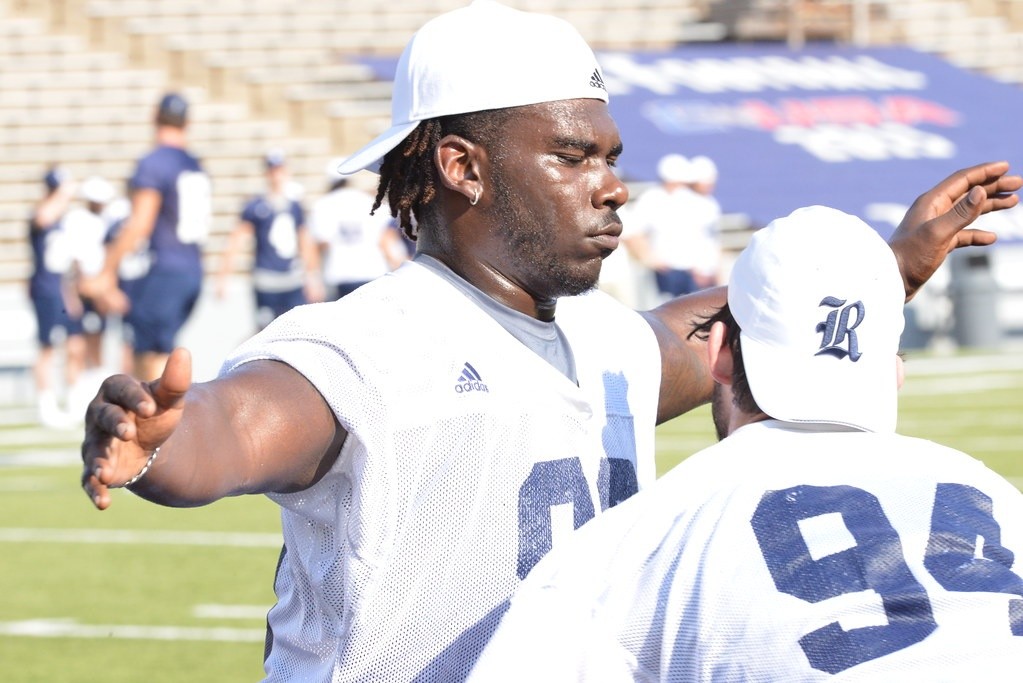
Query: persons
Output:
80,1,1023,683
461,203,1023,683
623,152,730,296
25,89,409,428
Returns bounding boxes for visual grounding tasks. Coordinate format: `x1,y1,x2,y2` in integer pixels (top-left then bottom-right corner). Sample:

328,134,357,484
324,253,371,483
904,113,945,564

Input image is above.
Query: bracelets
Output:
120,446,158,487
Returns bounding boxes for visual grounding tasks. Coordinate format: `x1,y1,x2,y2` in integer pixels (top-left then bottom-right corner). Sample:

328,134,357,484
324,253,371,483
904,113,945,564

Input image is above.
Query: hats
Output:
162,96,186,113
264,154,281,166
727,205,904,433
658,154,689,182
47,170,59,187
690,156,717,181
336,0,609,175
